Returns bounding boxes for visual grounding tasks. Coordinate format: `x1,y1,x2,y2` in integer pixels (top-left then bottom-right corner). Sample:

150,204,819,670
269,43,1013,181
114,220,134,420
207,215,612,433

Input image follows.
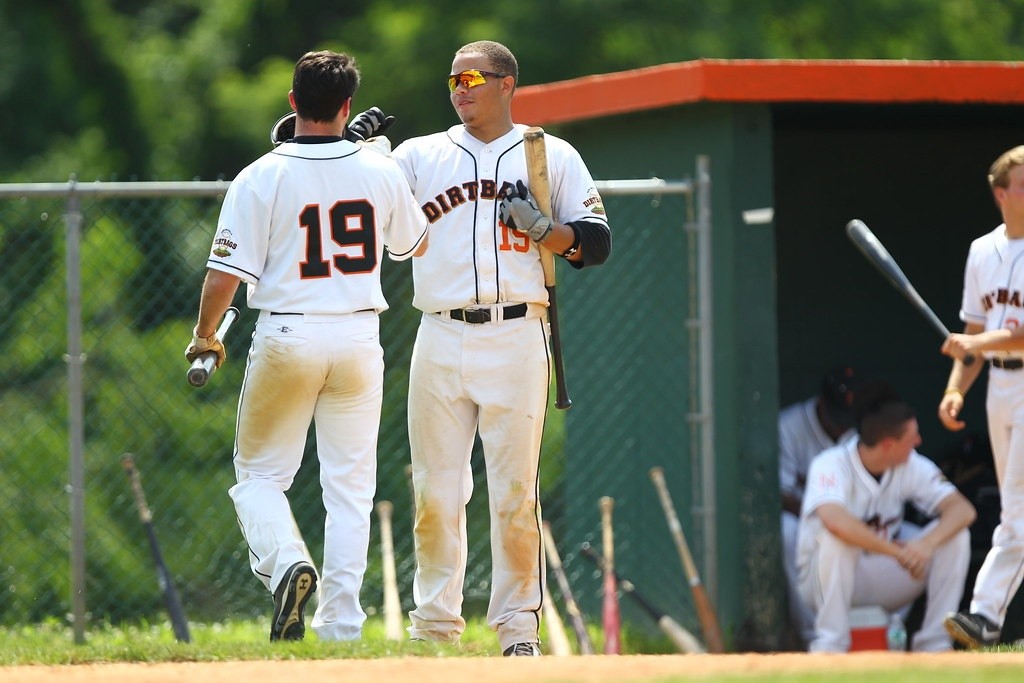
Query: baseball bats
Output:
374,499,406,639
187,304,240,387
523,127,574,411
846,216,976,370
540,464,727,659
119,452,190,643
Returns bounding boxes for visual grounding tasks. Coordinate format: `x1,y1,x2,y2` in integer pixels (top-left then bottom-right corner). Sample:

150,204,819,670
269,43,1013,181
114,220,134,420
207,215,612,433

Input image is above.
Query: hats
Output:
823,358,868,427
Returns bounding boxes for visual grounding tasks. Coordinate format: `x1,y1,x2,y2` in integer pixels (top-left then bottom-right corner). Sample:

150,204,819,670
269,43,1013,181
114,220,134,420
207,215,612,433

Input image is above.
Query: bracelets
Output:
943,388,965,398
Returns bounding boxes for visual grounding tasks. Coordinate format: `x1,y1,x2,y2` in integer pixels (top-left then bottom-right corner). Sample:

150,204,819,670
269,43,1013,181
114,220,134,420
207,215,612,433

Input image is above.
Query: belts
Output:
271,309,374,315
436,303,527,323
993,359,1023,369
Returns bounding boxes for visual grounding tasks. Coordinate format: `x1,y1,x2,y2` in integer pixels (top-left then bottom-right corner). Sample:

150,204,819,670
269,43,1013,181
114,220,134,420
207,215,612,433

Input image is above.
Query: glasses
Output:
448,69,506,92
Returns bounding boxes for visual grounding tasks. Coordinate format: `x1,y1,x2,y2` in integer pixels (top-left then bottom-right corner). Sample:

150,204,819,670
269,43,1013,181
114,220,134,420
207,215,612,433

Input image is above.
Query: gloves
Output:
496,179,551,242
185,325,226,372
347,107,395,140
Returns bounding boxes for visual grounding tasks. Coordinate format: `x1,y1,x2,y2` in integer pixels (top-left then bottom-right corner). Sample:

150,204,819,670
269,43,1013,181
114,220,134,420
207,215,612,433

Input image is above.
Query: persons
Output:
349,40,613,659
795,404,976,655
938,146,1024,649
185,48,432,641
776,364,915,647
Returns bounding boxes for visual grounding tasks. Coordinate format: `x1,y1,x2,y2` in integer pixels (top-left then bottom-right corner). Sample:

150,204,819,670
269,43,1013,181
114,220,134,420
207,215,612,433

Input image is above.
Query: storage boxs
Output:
849,607,891,648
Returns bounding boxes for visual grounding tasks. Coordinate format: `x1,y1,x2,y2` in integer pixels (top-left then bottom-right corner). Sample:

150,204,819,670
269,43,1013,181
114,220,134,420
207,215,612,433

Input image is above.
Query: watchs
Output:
556,222,580,258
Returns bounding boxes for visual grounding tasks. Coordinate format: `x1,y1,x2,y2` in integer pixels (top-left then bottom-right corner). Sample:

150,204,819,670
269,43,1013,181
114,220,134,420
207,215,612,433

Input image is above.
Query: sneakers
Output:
503,643,542,657
270,561,317,643
946,614,1000,648
